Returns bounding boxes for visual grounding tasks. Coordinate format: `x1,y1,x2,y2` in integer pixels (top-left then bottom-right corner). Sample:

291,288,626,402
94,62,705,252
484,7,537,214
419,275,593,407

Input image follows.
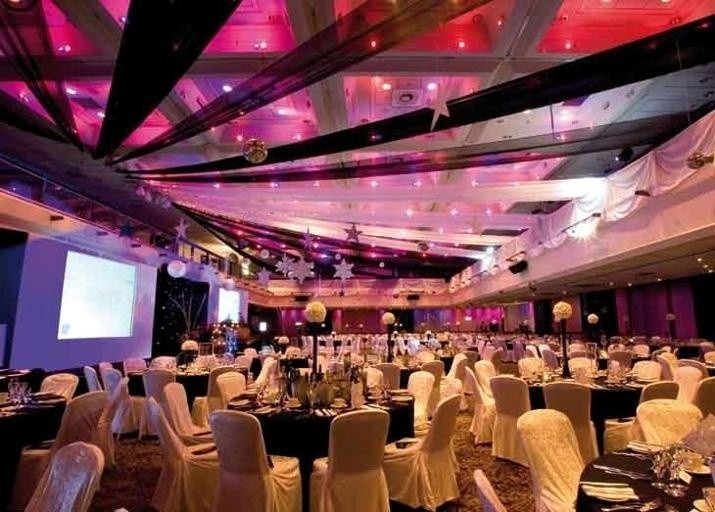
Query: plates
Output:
678,465,712,474
692,499,710,512
284,404,302,408
330,404,348,407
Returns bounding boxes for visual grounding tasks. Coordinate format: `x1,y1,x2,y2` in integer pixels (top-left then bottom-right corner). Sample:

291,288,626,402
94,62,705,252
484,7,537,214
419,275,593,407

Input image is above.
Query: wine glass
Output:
7,382,32,406
271,382,281,406
287,371,296,384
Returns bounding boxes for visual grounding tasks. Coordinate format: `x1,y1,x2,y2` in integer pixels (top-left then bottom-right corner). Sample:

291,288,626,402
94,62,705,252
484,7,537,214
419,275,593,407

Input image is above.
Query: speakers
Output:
508,259,528,274
295,296,309,302
407,294,420,300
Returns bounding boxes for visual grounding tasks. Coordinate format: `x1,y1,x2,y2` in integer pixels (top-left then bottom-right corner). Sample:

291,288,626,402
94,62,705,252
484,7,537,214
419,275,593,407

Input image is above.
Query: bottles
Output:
317,364,324,381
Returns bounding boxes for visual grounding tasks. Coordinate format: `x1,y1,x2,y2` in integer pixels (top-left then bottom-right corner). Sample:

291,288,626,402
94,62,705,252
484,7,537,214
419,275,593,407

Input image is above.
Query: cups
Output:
683,452,703,472
664,484,685,512
334,399,346,406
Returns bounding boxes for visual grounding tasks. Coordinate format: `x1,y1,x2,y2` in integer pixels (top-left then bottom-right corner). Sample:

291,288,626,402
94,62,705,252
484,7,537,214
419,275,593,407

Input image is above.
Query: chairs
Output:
517,409,586,512
473,469,508,512
383,393,463,512
147,393,219,512
309,409,391,512
22,441,105,511
209,410,304,512
